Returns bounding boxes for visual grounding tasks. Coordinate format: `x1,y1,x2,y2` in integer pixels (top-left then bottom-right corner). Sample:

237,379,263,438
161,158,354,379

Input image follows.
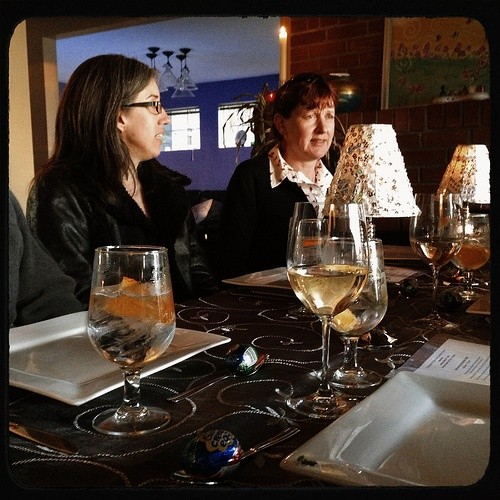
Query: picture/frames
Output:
381,17,491,110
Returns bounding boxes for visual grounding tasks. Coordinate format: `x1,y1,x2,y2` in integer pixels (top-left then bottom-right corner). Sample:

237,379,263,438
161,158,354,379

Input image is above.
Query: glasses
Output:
121,100,162,114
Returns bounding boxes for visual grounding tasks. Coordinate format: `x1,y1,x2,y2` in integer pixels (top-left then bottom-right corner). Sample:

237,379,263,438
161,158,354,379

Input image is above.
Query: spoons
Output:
169,354,269,403
169,427,301,487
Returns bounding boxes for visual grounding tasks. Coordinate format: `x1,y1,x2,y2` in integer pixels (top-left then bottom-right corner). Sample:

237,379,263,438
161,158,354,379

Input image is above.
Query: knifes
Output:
9,421,79,454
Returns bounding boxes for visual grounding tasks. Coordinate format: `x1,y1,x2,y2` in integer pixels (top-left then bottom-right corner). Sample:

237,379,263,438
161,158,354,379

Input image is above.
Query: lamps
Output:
328,73,362,113
322,123,422,284
146,47,198,98
434,144,492,286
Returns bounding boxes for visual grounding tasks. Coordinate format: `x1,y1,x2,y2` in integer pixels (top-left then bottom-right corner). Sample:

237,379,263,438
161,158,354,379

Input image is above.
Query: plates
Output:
220,267,291,291
9,310,232,406
278,371,489,486
467,293,490,315
383,245,422,259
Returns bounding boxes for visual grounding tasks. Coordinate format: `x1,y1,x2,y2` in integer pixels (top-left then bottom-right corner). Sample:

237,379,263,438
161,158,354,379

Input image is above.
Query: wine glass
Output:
326,238,390,387
287,201,367,418
409,192,466,330
450,212,488,299
286,218,329,320
87,245,175,437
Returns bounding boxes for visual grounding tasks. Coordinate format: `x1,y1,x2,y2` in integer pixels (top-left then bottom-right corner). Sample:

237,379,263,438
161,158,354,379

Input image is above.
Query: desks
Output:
8,259,490,490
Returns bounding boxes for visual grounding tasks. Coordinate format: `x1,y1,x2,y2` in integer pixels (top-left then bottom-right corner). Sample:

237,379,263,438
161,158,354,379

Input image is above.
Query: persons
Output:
28,54,218,304
9,192,82,325
221,74,341,275
